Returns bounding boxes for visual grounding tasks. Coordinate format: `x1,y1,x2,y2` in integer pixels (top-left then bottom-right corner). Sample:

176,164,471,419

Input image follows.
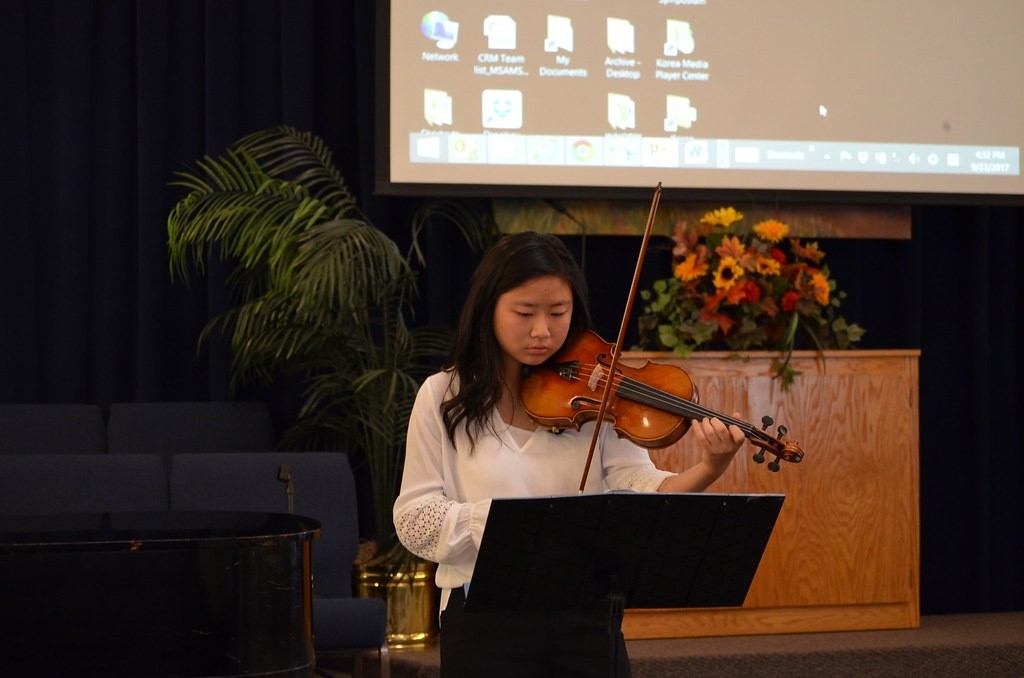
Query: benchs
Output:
0,405,276,453
0,452,390,678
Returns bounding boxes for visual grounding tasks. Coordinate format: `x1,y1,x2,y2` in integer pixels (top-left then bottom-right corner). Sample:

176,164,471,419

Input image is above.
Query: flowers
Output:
638,206,865,392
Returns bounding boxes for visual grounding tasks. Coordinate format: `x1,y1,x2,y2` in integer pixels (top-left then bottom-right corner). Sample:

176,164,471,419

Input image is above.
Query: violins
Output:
516,325,806,472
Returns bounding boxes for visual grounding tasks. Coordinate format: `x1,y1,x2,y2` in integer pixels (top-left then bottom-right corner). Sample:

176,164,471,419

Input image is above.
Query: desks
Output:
0,512,326,677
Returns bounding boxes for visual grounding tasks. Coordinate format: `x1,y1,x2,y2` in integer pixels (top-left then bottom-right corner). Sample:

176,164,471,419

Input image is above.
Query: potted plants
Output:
166,125,484,648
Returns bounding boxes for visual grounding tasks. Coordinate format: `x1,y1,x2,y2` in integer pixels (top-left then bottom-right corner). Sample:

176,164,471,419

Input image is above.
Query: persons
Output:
393,230,747,678
